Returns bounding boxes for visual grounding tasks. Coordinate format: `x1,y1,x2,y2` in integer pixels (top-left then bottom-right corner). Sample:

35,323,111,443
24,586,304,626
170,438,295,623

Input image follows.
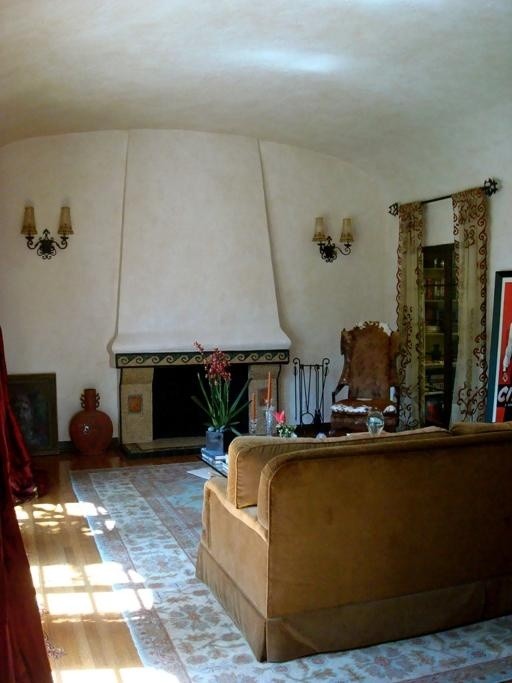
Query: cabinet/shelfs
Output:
422,243,458,397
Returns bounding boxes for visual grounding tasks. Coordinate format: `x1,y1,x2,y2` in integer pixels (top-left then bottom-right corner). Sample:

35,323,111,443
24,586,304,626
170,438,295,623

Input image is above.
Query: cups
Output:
365,412,385,437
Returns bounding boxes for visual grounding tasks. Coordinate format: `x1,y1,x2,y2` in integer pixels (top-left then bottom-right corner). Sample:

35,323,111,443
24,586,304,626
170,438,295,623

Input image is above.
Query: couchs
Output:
195,421,512,663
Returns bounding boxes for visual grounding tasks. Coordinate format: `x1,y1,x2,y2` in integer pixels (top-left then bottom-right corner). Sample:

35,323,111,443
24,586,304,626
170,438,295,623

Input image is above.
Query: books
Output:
424,278,445,298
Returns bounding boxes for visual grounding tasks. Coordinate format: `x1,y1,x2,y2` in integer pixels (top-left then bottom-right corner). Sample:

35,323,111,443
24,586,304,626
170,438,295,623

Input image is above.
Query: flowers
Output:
191,340,252,436
272,411,297,438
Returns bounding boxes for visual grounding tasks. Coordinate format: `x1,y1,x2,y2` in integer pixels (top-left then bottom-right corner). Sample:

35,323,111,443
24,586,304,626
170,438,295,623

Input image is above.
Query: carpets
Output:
70,461,512,683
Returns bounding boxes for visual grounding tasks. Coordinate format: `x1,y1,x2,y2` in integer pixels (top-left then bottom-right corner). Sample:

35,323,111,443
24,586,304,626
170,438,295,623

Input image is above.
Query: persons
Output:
501,321,511,423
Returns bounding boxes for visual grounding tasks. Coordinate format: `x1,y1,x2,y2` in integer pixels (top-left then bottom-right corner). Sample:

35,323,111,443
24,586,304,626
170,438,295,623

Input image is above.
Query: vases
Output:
69,389,113,470
205,431,235,455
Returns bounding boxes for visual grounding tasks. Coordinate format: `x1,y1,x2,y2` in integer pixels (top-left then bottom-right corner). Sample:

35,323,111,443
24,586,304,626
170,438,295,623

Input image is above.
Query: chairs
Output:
330,320,402,436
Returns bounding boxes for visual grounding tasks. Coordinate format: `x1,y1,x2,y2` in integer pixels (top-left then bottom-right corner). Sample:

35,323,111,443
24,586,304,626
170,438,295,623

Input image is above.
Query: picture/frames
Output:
483,270,512,423
8,373,58,451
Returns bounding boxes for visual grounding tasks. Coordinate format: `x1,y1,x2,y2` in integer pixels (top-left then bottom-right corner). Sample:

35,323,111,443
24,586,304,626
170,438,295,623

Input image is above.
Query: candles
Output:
267,371,272,407
251,392,257,422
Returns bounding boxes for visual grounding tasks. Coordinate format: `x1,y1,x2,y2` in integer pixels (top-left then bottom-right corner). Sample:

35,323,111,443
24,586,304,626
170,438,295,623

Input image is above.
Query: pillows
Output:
449,420,512,436
228,425,454,510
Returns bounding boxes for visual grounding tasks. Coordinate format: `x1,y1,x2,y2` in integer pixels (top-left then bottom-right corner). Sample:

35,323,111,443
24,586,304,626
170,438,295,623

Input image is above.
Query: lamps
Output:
21,207,75,260
312,217,354,263
